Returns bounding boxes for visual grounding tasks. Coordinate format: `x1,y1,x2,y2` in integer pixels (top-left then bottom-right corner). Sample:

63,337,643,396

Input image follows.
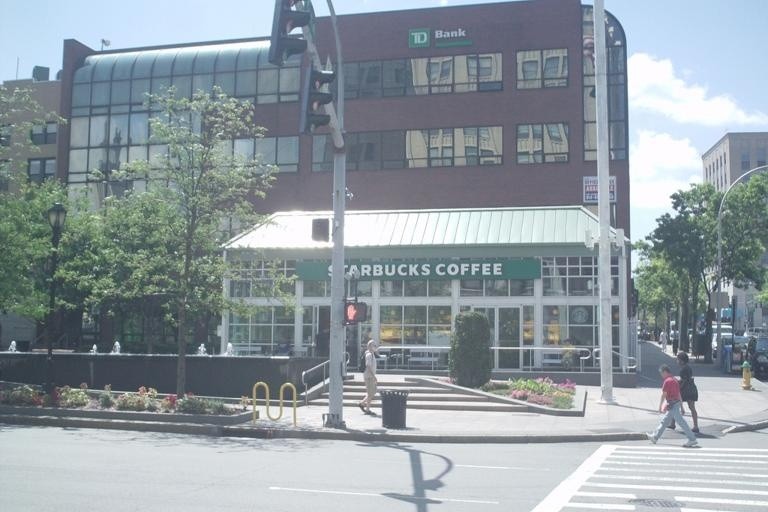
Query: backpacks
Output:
358,351,373,373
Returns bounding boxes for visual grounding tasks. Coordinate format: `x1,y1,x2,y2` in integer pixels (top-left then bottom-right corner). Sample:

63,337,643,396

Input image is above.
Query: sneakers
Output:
359,403,376,415
645,425,699,447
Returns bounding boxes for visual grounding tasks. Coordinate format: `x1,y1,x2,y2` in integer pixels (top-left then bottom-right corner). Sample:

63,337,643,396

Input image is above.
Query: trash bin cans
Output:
382,391,407,429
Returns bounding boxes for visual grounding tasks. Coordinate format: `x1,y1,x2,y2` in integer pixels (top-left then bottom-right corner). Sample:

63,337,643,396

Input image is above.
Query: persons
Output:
358,339,381,416
666,349,701,433
669,325,679,354
313,325,330,358
646,364,698,447
659,327,669,353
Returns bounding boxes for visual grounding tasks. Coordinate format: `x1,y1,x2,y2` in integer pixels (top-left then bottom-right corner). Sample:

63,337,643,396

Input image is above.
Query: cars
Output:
712,324,767,379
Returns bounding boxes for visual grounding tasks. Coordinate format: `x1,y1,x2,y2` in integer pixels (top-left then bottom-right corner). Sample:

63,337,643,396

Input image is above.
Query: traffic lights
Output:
269,0,312,68
298,47,336,137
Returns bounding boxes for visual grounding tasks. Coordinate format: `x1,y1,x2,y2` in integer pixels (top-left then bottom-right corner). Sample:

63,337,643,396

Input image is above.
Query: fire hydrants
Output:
739,359,753,390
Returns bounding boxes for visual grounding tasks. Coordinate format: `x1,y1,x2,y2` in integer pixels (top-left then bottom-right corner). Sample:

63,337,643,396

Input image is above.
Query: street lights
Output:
44,202,67,394
